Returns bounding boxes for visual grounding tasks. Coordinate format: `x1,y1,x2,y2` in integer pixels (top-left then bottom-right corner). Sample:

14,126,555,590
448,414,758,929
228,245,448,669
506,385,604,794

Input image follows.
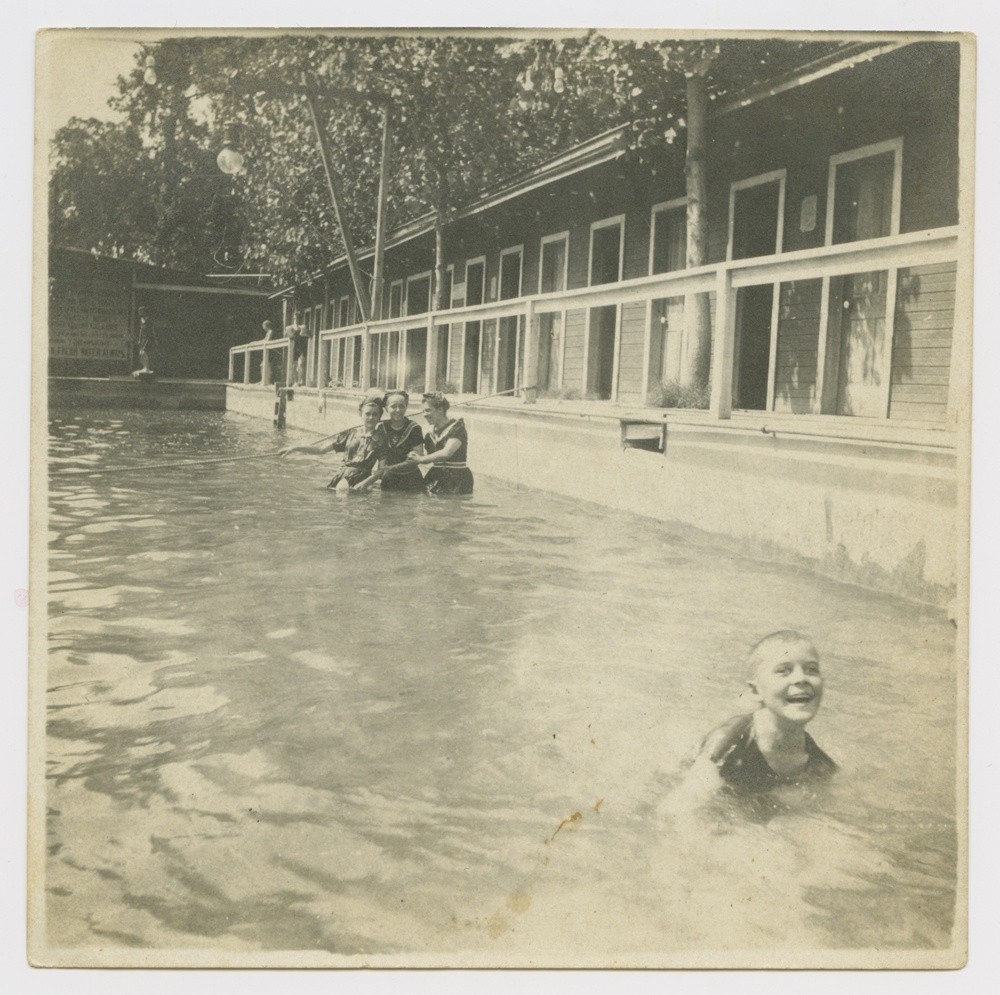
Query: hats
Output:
382,390,409,408
358,396,383,417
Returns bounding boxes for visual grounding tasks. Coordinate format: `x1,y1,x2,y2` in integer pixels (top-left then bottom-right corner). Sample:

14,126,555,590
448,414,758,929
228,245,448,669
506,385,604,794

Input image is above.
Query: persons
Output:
249,320,275,386
405,390,473,496
286,323,310,376
136,305,160,373
375,390,425,492
685,628,839,816
278,395,389,495
